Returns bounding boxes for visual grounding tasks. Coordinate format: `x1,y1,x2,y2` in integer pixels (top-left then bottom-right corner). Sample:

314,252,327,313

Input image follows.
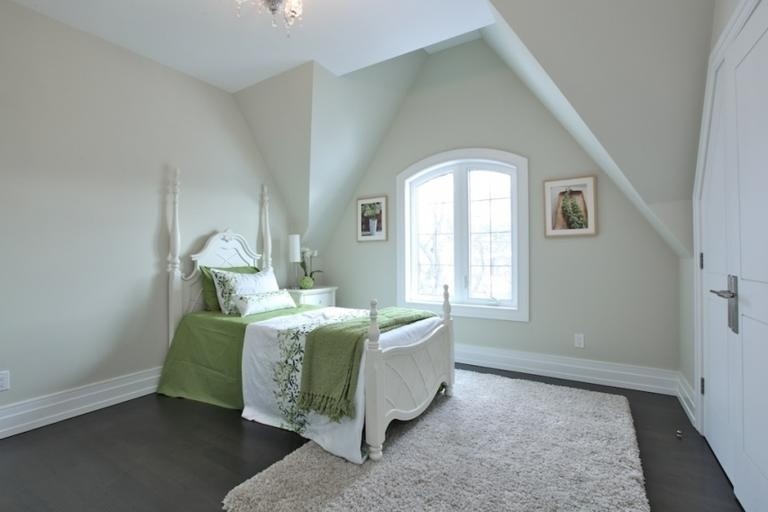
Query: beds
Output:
167,225,456,462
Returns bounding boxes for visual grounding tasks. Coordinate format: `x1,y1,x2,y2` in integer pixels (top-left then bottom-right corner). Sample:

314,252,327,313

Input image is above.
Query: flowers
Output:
297,246,324,281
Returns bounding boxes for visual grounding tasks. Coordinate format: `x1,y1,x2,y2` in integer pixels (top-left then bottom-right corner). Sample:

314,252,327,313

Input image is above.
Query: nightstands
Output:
288,286,337,307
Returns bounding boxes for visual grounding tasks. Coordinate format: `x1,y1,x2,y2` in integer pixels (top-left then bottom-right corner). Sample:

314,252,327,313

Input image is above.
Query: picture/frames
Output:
355,194,388,243
541,174,598,239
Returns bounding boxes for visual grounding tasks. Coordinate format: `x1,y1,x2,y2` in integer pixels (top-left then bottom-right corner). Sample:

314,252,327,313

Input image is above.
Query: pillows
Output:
199,263,298,320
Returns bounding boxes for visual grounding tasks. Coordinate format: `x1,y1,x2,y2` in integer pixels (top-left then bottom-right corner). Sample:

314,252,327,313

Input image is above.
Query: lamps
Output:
286,232,300,263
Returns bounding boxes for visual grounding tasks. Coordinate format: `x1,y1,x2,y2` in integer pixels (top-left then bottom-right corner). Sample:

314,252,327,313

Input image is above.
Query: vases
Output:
299,275,313,288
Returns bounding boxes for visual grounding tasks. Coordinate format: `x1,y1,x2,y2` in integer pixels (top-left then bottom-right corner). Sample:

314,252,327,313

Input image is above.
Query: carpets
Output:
221,366,651,512
